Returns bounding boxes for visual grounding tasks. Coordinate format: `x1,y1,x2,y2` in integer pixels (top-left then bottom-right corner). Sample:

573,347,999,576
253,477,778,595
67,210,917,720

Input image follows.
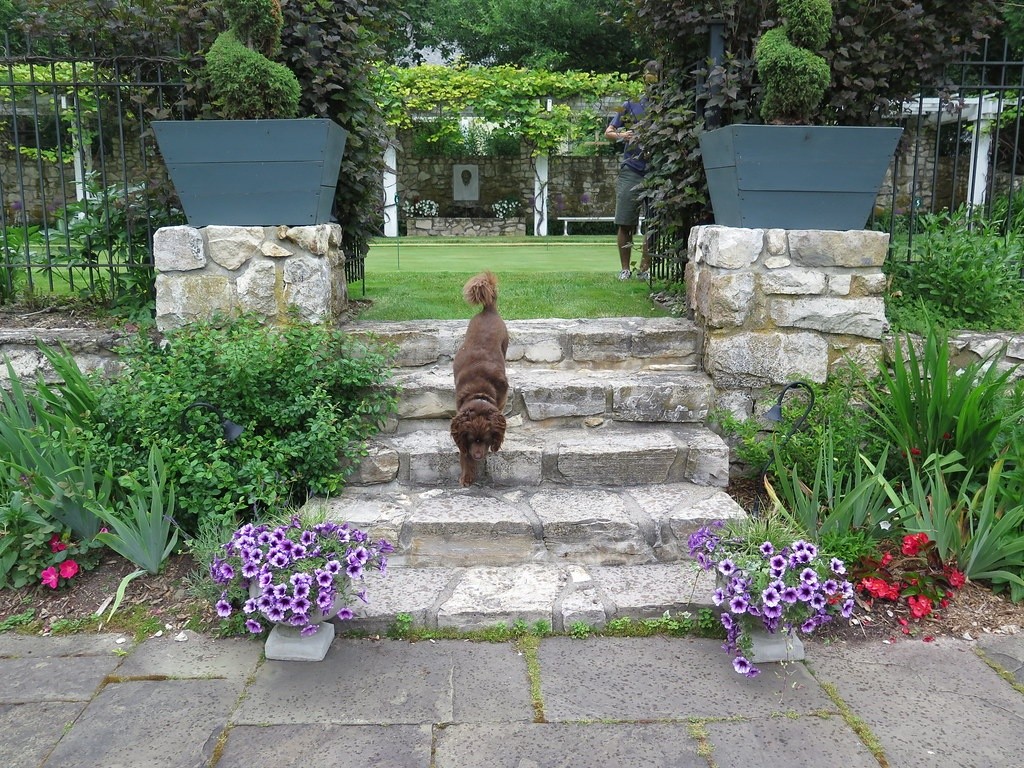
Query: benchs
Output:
557,216,646,236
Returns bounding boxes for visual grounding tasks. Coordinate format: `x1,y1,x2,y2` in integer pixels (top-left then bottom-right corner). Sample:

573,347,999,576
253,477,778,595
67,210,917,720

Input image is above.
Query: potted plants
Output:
149,0,350,227
697,0,906,232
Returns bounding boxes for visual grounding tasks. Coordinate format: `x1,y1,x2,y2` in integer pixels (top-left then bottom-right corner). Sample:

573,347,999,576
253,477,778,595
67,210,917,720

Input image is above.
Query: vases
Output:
247,569,350,626
714,570,763,619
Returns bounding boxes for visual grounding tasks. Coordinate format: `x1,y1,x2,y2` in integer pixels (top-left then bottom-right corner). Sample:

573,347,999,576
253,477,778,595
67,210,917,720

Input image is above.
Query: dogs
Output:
450,268,509,488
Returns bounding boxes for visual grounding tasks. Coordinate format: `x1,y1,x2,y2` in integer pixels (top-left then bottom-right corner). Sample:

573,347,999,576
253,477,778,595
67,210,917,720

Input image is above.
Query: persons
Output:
604,60,660,282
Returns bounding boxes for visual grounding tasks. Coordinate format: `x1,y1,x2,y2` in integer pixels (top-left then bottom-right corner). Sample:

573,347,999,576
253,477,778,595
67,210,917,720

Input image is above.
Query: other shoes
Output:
636,271,650,280
618,270,631,280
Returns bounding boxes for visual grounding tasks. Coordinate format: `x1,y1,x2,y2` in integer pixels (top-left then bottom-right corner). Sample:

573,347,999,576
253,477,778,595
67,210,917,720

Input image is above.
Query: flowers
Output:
691,520,854,677
208,480,392,636
